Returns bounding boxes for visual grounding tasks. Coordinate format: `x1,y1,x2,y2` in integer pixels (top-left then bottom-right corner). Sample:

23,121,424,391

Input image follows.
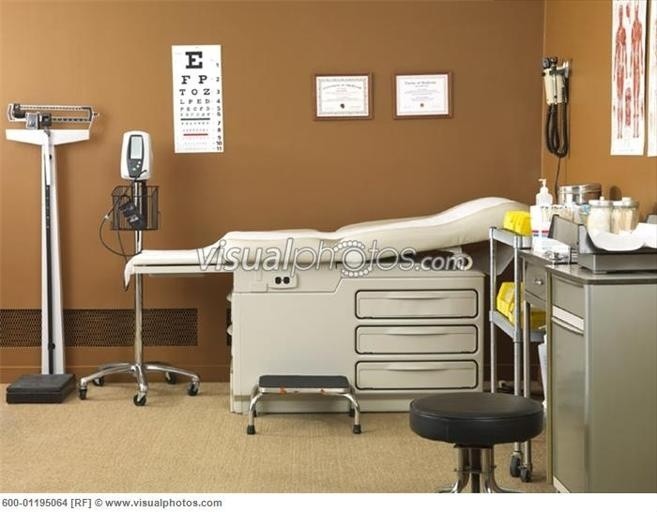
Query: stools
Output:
405,390,549,492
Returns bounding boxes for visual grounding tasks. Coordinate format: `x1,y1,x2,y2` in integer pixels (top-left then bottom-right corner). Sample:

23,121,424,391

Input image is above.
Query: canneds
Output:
585,200,640,235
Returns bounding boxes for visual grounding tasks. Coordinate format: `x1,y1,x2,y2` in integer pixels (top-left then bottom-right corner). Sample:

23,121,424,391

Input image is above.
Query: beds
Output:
121,194,525,289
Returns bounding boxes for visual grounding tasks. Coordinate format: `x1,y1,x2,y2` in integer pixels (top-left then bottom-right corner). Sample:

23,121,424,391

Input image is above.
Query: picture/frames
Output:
310,69,455,123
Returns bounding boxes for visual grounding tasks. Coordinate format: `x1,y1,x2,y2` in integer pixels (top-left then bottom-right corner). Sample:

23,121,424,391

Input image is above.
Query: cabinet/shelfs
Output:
227,267,491,417
489,226,656,490
